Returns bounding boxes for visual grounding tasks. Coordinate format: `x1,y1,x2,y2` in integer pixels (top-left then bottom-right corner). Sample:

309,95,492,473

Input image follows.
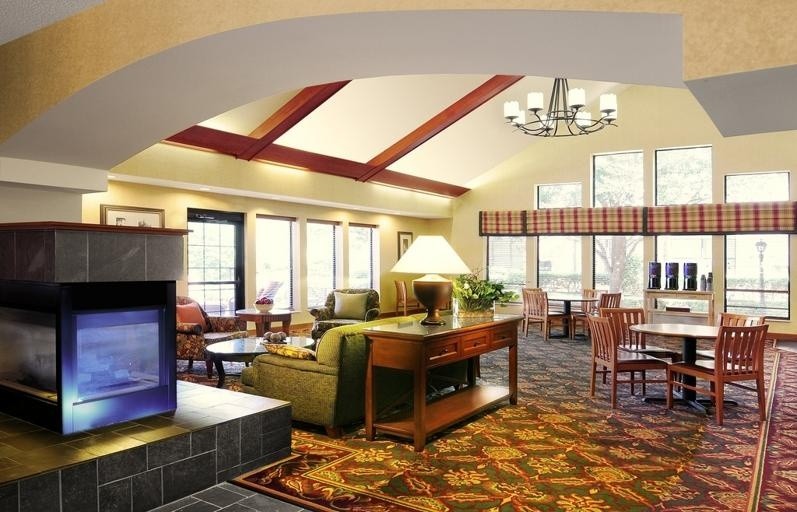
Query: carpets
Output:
177,315,797,512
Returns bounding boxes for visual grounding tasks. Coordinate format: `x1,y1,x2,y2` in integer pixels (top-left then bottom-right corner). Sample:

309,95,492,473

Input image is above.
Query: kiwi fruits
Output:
263,332,287,342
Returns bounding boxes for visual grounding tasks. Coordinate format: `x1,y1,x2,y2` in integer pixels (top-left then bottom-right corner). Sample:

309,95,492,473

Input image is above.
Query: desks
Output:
232,309,291,337
361,313,524,451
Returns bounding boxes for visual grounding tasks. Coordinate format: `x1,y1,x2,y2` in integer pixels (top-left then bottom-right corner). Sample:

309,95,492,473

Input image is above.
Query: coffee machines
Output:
664,261,679,289
648,261,662,288
684,263,699,291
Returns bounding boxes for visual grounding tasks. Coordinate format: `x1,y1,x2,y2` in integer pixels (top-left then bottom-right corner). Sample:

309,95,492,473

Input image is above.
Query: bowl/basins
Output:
253,303,274,313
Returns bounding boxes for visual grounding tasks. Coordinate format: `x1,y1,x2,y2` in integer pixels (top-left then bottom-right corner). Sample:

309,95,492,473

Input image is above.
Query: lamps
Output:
504,79,618,136
388,232,473,324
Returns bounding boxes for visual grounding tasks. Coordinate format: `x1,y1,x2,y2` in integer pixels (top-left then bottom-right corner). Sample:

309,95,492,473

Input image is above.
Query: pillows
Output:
263,342,315,362
175,305,205,330
332,290,369,321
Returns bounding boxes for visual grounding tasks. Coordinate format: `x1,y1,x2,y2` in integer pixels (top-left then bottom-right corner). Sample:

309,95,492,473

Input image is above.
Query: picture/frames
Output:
395,231,414,262
97,203,166,230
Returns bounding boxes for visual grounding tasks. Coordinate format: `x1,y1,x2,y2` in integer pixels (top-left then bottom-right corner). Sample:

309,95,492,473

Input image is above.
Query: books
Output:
665,306,690,312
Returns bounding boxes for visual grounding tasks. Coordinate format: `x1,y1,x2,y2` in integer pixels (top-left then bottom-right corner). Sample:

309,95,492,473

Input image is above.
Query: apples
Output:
256,297,274,304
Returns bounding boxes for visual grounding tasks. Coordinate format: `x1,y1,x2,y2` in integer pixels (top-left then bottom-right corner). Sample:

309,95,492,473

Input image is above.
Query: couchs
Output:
248,307,479,437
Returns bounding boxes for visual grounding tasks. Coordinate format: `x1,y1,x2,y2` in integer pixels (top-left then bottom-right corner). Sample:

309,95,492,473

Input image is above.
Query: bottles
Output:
701,271,713,291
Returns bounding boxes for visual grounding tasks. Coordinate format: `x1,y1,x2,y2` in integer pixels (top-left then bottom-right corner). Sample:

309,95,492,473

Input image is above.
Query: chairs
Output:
174,293,241,374
518,286,768,429
394,277,417,314
311,286,380,338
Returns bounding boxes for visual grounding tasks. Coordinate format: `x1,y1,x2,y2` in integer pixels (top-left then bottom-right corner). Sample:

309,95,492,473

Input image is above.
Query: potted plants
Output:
450,276,516,317
